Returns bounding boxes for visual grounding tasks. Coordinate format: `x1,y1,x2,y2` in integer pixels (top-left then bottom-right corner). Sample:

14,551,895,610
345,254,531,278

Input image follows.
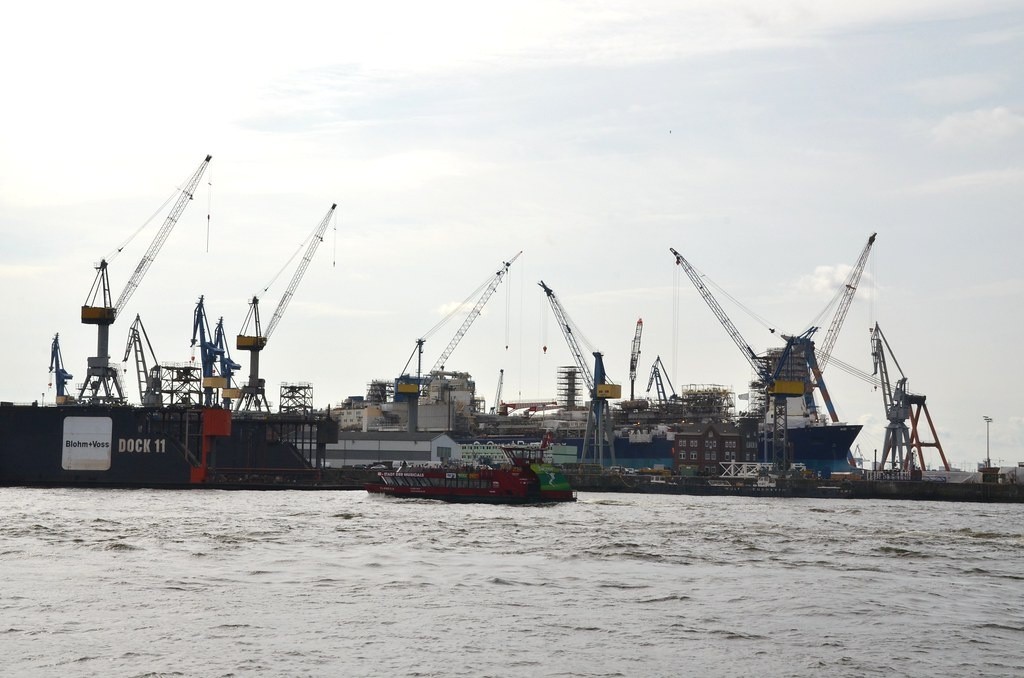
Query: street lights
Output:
982,415,993,468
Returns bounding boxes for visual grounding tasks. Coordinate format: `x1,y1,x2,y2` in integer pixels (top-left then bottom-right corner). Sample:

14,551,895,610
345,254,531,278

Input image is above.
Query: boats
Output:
271,364,579,468
365,431,580,506
0,359,369,491
452,347,866,478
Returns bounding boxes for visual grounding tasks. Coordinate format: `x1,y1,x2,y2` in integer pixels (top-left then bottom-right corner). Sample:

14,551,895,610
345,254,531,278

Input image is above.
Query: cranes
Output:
72,153,340,413
535,231,952,479
390,249,524,403
